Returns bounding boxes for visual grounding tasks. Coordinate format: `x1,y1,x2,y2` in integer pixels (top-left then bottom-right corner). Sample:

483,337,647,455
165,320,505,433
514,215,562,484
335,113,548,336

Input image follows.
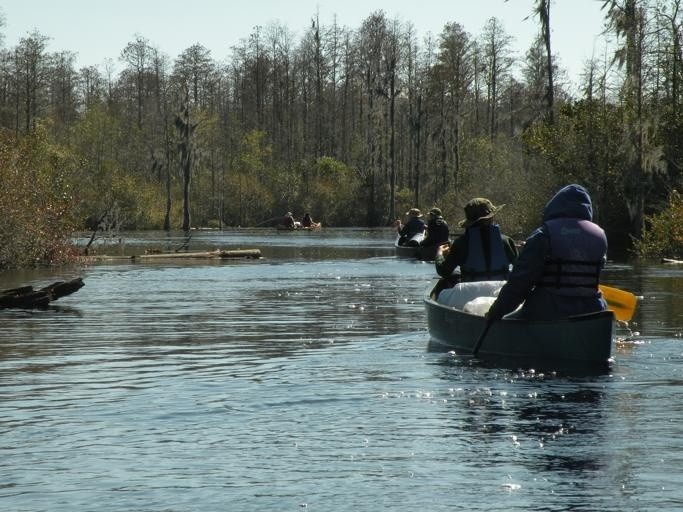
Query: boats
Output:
423,260,615,376
394,230,457,260
277,221,324,236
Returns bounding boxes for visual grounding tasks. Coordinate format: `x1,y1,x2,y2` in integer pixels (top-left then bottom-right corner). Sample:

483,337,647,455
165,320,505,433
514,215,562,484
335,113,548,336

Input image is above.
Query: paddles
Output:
597,282,639,323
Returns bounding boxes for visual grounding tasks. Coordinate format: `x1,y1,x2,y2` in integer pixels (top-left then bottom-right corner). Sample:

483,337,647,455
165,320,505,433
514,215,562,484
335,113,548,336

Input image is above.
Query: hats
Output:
405,208,423,218
428,207,444,219
457,197,506,228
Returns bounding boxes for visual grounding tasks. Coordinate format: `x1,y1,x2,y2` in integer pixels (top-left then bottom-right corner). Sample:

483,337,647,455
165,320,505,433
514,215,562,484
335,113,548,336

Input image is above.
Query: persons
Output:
483,183,608,320
396,207,449,247
435,197,519,288
284,212,317,227
